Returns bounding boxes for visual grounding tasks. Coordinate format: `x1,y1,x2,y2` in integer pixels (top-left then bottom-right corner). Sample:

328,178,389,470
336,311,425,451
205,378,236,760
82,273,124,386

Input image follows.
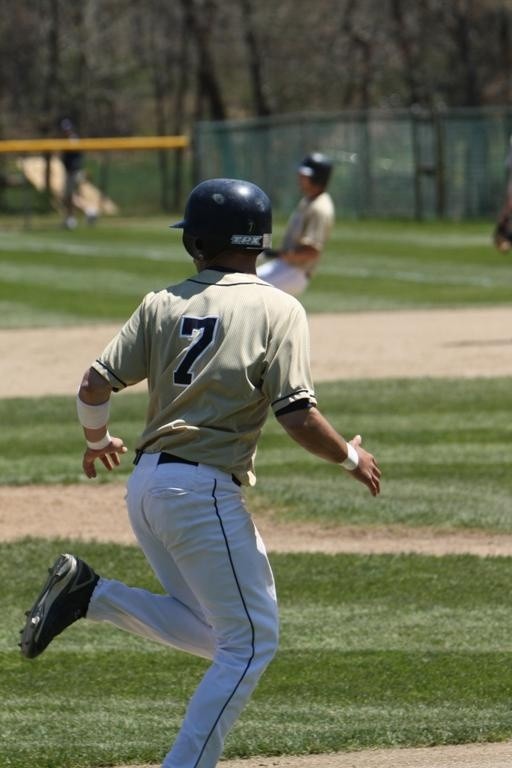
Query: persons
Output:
255,151,336,294
20,178,385,768
57,117,99,230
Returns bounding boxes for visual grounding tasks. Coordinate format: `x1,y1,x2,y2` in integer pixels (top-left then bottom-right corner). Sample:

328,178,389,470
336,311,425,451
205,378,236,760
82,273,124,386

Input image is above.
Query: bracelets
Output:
86,430,113,451
339,441,362,471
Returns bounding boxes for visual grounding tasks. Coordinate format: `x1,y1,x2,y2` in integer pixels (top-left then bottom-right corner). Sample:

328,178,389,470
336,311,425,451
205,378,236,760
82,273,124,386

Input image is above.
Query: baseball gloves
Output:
494,215,511,252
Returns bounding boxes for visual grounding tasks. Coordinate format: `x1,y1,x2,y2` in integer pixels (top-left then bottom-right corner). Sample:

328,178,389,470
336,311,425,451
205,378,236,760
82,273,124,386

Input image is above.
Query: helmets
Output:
299,150,334,187
169,179,273,252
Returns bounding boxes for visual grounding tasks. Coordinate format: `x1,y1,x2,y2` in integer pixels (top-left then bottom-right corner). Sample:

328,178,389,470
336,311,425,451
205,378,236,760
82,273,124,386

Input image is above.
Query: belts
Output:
133,449,242,486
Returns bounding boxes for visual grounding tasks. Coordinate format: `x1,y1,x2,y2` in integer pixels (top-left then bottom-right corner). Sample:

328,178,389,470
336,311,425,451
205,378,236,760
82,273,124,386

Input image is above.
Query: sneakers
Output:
17,552,100,659
